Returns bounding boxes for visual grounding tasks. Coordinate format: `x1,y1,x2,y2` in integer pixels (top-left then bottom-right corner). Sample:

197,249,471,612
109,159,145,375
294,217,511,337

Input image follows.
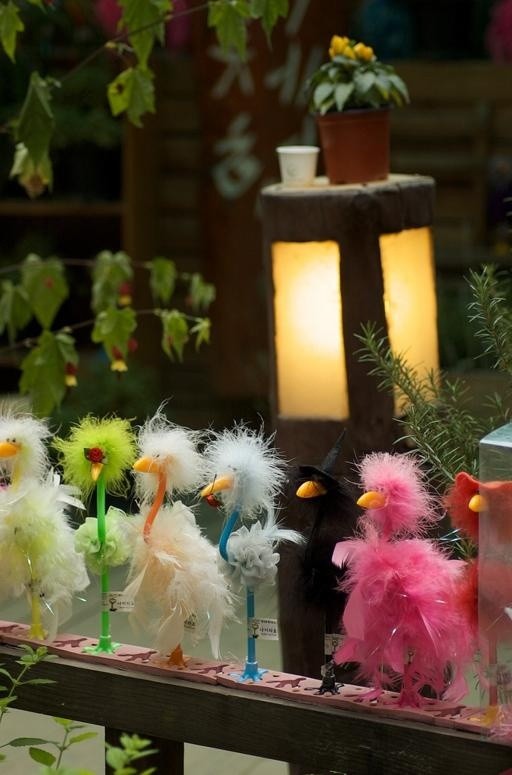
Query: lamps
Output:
262,172,445,681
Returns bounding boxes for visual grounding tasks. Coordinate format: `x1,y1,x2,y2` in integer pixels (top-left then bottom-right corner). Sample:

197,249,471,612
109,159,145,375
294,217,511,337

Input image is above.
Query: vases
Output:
315,107,391,184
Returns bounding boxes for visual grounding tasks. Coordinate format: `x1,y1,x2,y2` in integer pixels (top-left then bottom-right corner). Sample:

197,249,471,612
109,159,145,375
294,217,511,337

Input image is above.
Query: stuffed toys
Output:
0,403,512,722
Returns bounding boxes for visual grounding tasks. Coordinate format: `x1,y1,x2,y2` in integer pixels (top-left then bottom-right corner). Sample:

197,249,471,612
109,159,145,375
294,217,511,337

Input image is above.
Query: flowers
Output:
302,33,411,116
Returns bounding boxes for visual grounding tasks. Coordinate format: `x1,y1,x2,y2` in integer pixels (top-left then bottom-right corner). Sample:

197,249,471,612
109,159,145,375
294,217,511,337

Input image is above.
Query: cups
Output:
277,144,319,186
